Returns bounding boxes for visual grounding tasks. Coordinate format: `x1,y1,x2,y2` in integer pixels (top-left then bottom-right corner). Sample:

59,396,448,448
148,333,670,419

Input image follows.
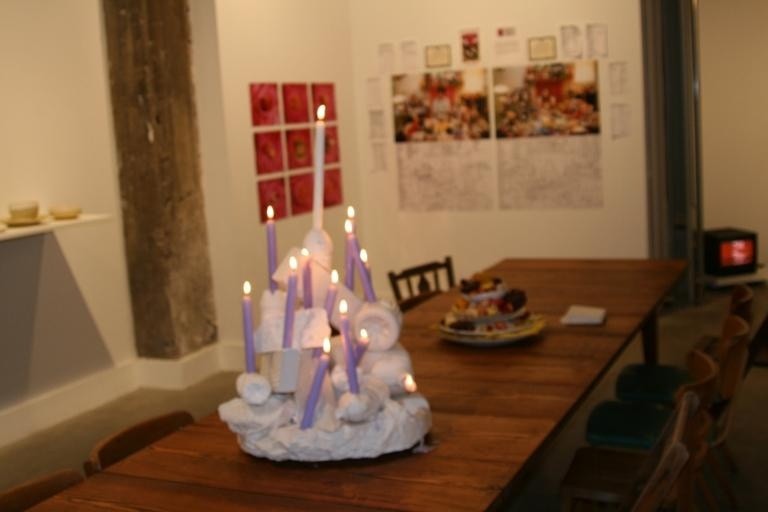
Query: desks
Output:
24,257,695,512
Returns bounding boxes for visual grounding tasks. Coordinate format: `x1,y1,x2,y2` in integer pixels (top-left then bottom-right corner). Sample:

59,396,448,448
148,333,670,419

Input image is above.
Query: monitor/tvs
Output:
704,227,760,278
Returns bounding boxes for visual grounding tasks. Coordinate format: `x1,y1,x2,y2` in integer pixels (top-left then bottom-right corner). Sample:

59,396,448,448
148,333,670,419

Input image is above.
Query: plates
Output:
2,218,43,226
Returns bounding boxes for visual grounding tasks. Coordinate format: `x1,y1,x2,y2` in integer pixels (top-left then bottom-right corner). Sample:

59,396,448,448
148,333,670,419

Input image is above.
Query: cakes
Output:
217,226,432,462
437,275,531,335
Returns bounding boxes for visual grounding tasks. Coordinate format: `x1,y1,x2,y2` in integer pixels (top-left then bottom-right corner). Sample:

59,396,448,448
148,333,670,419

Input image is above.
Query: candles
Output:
341,217,378,302
351,326,372,368
322,270,339,326
335,302,362,397
267,205,279,292
239,280,259,374
307,103,330,229
342,202,360,290
280,254,303,347
298,335,332,430
300,248,318,312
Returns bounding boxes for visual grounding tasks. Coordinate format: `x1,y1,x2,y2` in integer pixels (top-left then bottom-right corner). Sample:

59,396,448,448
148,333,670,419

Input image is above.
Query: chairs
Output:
387,257,461,308
1,468,86,511
494,279,754,511
83,404,195,477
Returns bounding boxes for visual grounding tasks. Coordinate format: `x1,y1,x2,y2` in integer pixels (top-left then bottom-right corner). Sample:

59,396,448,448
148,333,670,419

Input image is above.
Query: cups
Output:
9,202,37,220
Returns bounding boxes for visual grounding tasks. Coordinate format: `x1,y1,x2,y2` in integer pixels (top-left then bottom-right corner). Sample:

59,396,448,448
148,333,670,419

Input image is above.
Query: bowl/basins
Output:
49,207,82,218
